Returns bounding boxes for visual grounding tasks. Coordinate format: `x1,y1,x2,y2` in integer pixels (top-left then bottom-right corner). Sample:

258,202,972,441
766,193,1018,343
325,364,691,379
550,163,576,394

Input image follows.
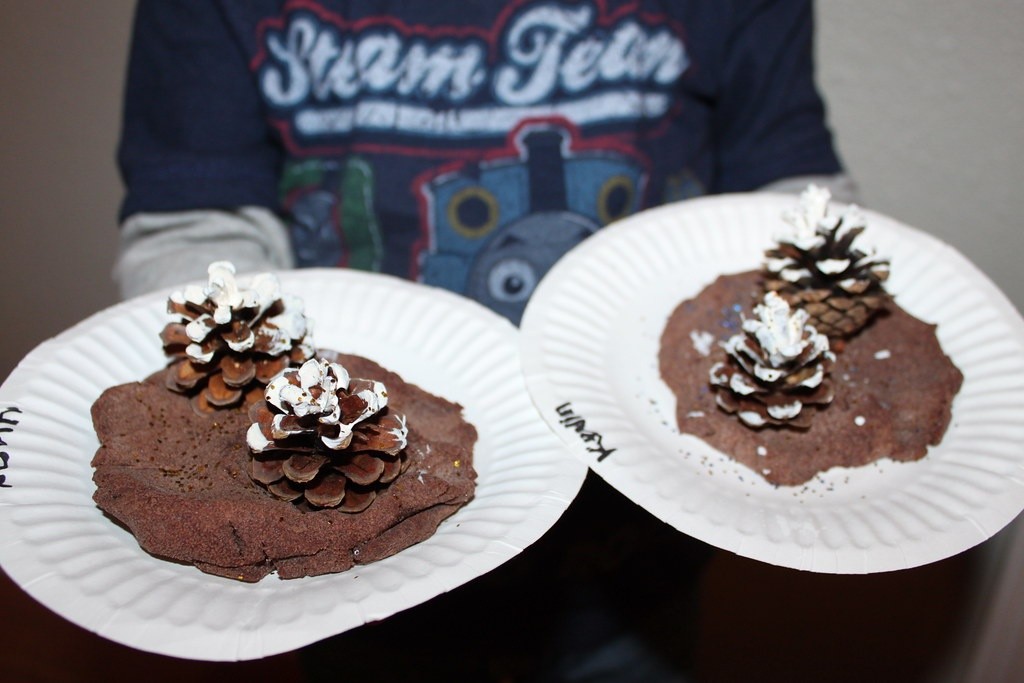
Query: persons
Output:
114,0,843,683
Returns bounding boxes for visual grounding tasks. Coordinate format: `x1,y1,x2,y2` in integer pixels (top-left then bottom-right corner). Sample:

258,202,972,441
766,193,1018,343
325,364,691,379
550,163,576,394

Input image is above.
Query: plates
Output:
0,267,588,662
518,192,1024,575
